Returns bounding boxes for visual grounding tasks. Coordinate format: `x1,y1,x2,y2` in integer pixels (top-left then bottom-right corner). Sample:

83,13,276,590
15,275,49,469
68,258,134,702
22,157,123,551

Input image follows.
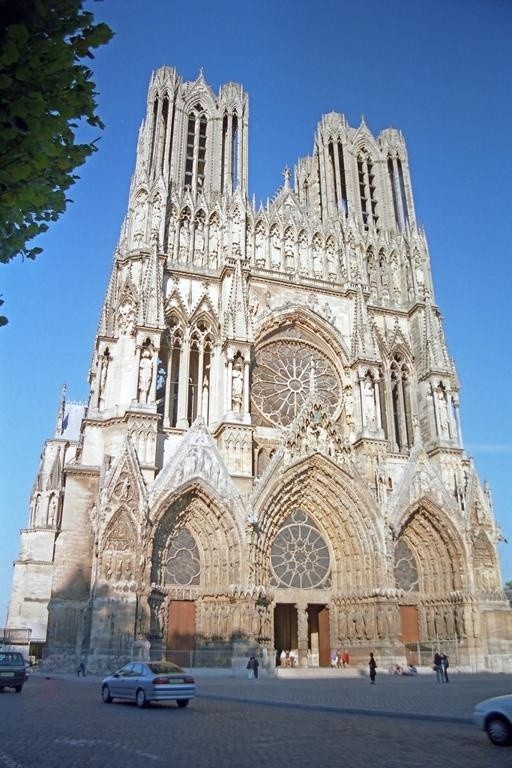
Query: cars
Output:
0,651,29,693
100,661,197,708
472,692,512,748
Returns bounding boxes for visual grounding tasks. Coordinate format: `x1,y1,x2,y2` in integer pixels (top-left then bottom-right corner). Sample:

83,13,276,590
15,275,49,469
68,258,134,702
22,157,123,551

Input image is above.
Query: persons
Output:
391,664,402,676
246,656,254,680
138,349,155,405
365,376,375,428
279,649,287,668
251,657,260,680
403,663,418,676
440,653,449,683
433,652,445,684
335,648,342,669
368,652,377,684
341,649,350,667
76,650,86,677
275,647,281,667
228,361,244,412
98,354,107,392
289,649,296,667
